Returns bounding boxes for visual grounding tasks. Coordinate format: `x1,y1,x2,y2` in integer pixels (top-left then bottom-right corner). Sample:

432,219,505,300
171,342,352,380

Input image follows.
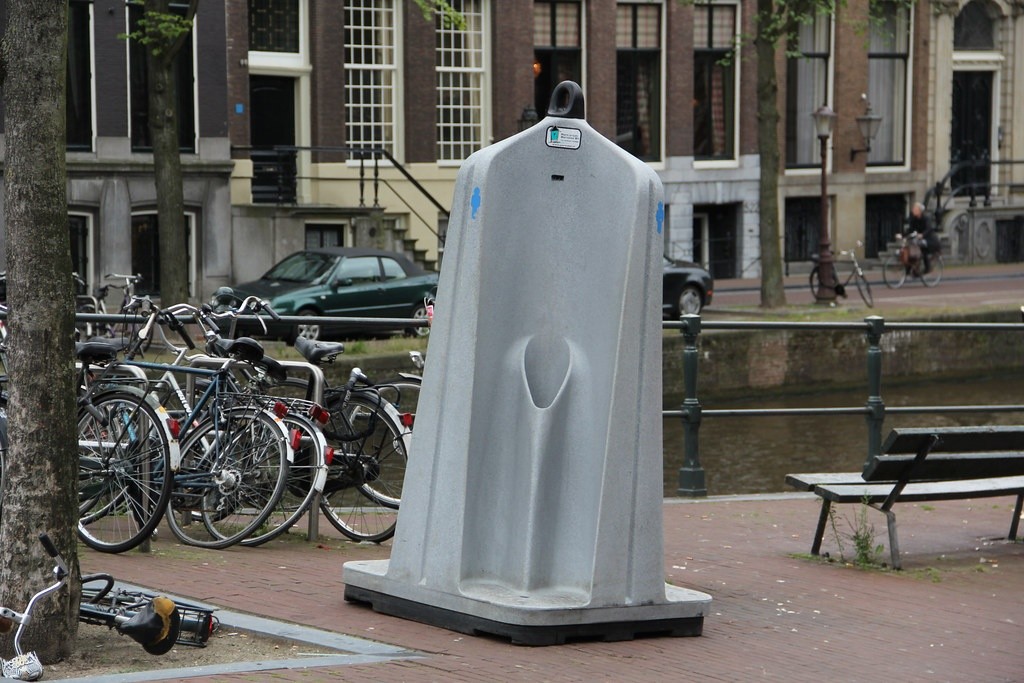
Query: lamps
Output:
849,92,883,163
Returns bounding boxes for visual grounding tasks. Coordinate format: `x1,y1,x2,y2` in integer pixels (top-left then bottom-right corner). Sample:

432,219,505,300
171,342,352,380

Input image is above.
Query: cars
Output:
214,244,450,347
663,253,715,319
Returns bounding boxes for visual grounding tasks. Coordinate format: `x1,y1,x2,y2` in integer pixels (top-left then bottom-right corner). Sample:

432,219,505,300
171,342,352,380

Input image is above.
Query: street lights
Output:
812,102,838,303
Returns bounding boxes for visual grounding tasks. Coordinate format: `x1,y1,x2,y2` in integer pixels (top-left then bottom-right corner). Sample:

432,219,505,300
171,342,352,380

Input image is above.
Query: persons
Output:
905,201,942,276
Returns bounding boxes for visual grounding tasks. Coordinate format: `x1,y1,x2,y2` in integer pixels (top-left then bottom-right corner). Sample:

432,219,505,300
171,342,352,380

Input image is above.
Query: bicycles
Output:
3,528,217,678
808,240,873,309
884,231,946,287
2,270,411,558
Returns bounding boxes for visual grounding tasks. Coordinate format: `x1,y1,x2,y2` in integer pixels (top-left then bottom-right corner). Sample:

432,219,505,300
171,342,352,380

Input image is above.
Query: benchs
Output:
785,425,1024,571
